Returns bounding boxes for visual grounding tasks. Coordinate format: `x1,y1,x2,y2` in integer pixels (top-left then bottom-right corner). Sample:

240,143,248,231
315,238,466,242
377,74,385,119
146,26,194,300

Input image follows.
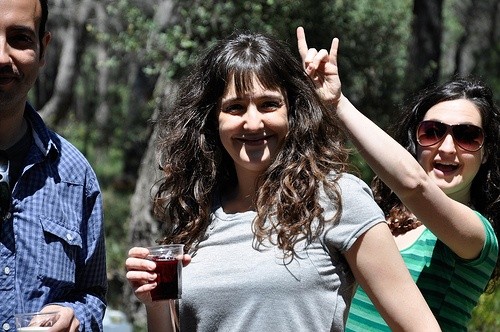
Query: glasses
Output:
416,120,486,152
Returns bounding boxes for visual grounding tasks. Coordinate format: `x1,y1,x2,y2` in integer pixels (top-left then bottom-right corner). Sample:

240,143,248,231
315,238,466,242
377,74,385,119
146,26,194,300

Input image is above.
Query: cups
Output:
14,311,58,332
144,243,185,302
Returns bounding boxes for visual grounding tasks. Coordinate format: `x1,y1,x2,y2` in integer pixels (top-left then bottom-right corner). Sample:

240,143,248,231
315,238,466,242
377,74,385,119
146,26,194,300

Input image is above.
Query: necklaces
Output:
237,193,252,199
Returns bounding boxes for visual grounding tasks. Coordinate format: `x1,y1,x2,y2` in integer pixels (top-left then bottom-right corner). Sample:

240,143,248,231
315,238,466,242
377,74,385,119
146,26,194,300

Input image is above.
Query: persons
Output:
124,31,443,332
294,23,500,332
0,0,107,332
